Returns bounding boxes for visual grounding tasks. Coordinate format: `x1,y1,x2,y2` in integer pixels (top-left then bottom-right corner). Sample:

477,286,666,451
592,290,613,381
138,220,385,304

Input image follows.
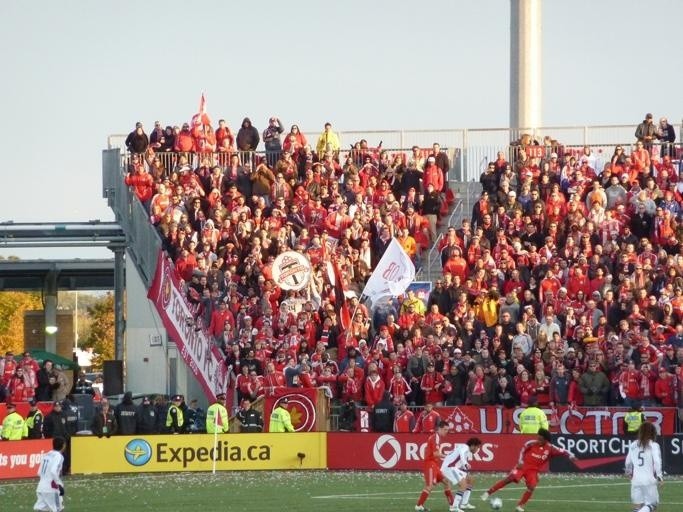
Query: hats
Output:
7,392,293,412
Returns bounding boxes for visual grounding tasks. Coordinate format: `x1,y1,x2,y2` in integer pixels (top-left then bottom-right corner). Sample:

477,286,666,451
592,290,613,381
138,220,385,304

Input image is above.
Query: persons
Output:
32,436,68,512
439,437,481,512
0,350,120,438
623,422,664,512
414,419,461,511
479,427,580,512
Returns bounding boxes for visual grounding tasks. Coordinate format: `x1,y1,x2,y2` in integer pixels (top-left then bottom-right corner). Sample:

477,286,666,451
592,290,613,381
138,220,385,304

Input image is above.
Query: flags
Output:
190,96,206,127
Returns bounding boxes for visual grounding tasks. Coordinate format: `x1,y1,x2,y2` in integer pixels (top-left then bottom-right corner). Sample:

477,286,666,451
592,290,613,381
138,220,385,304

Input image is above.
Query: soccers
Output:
491,497,502,508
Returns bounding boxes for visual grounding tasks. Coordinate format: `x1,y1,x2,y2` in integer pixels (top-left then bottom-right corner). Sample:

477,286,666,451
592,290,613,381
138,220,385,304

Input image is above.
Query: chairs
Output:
406,185,456,265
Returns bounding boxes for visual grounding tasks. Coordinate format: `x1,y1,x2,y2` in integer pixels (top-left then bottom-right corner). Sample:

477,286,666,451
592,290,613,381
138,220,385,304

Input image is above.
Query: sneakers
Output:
479,491,490,501
448,503,478,512
413,503,432,512
513,506,526,512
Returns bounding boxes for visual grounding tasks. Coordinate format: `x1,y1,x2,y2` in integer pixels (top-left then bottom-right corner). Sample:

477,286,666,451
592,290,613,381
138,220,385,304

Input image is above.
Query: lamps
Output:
40,261,59,335
